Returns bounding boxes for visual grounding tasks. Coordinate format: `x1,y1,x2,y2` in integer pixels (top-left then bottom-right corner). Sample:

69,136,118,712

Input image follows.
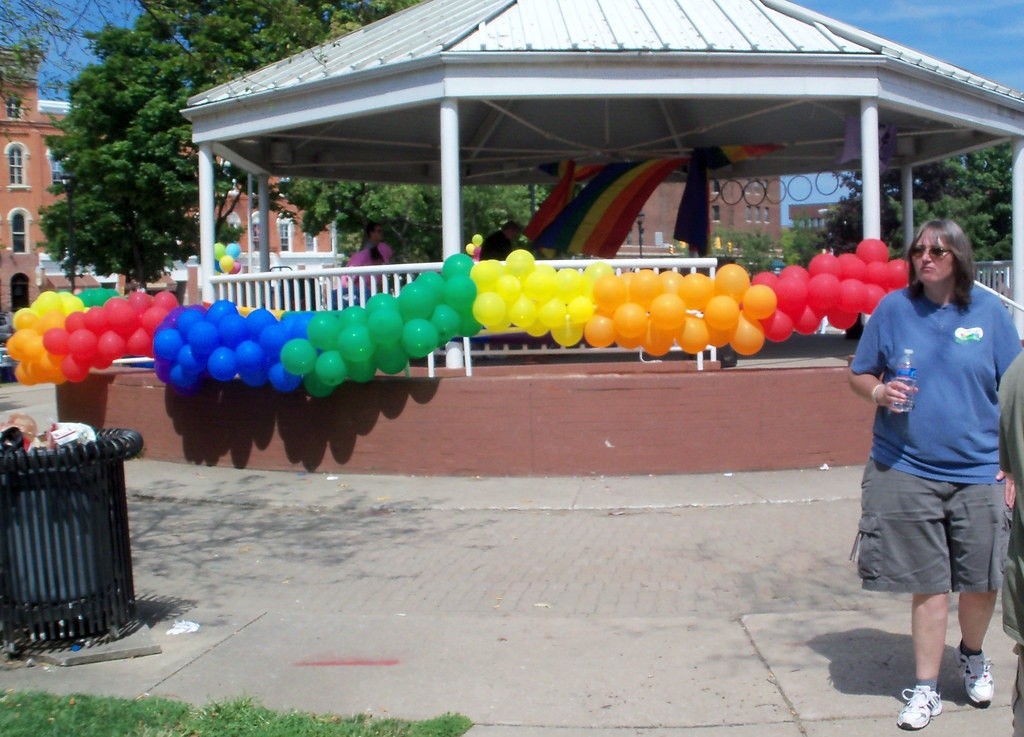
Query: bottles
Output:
892,349,915,413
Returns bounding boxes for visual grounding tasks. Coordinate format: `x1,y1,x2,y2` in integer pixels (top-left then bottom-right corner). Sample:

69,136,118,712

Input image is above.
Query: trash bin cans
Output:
0,428,144,646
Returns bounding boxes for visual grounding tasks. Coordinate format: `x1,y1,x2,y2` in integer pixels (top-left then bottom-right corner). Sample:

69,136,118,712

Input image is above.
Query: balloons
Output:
5,233,911,398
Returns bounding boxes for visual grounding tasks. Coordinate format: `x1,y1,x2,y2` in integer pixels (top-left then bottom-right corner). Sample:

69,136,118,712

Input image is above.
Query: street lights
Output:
60,173,79,291
636,210,644,260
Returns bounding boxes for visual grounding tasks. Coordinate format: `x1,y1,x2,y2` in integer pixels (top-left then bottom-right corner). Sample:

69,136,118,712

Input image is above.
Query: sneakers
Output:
953,644,994,709
896,685,942,730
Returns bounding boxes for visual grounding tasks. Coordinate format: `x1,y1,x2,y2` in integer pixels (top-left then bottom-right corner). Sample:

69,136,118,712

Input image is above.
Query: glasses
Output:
910,247,952,257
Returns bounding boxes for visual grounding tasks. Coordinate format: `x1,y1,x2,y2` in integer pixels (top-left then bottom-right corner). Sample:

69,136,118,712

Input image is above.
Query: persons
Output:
365,222,385,249
847,218,1023,730
998,347,1024,737
479,221,521,261
342,238,396,285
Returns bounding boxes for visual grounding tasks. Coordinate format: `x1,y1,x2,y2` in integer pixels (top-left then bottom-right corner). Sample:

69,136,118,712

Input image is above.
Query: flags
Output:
836,112,898,176
520,142,786,260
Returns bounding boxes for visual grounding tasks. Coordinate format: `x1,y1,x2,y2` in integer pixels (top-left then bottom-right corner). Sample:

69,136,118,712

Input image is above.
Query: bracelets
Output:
872,384,884,406
